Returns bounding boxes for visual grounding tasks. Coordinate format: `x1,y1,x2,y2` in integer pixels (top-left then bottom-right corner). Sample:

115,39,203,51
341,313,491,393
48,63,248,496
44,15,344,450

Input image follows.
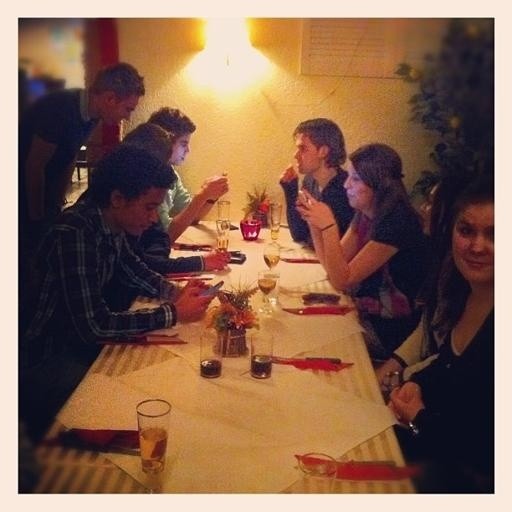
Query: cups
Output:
250,332,273,380
199,329,223,378
298,451,337,494
239,218,263,240
136,399,171,477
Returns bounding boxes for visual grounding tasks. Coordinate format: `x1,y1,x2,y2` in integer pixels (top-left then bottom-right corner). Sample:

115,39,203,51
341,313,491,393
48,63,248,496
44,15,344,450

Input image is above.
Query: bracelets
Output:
206,198,216,204
376,370,401,384
320,222,338,231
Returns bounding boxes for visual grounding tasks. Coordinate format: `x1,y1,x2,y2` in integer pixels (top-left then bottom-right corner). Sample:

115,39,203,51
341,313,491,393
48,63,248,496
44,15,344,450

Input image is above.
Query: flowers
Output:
240,185,274,219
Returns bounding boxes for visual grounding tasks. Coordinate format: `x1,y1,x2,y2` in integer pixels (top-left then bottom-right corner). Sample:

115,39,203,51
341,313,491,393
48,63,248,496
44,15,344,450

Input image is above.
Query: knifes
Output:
96,335,185,344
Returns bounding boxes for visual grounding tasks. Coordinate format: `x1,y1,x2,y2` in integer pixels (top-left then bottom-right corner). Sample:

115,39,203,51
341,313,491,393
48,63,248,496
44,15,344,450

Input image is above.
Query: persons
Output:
122,123,232,276
20,59,145,216
278,119,355,243
19,144,218,420
291,142,433,359
150,106,229,244
375,165,483,395
385,178,494,492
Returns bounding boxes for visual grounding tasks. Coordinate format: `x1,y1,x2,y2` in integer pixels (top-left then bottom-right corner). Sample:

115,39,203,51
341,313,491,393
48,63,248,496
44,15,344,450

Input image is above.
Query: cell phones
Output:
231,253,246,264
199,281,224,296
298,190,310,219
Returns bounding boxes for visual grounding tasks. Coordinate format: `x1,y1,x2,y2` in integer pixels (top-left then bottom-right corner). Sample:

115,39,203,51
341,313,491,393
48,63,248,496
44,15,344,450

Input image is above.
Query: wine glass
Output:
214,201,231,273
270,203,282,245
257,247,280,313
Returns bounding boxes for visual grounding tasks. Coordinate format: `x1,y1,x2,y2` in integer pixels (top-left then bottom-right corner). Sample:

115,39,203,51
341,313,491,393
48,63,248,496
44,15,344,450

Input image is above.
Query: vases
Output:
252,213,267,228
